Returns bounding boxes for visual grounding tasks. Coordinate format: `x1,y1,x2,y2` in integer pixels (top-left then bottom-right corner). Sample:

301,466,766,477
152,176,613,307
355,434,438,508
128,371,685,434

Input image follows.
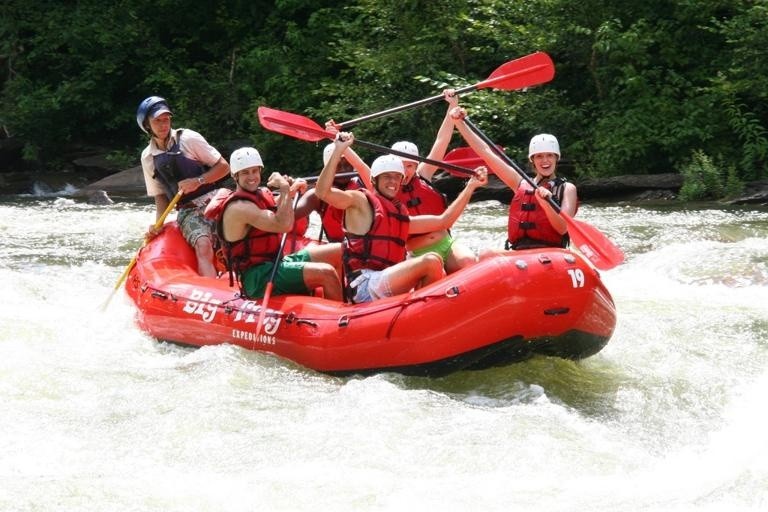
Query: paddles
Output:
257,107,488,183
291,140,506,185
256,190,300,345
453,110,626,271
330,51,556,130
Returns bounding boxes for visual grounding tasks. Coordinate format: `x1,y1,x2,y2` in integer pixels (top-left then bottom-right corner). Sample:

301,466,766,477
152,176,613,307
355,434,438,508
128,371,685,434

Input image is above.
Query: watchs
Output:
197,174,209,186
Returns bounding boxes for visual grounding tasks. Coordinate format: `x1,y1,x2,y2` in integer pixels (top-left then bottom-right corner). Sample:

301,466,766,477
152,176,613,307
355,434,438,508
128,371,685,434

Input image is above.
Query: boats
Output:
124,209,617,380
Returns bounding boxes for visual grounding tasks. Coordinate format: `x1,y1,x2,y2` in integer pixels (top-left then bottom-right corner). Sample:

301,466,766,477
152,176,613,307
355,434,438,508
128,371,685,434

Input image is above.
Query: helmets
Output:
136,95,167,134
229,147,264,175
390,141,419,164
528,133,560,163
323,141,344,167
370,154,405,180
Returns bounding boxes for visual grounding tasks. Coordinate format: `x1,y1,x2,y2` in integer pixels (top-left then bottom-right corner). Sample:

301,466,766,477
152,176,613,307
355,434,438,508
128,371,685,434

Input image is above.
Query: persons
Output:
322,84,467,278
311,129,491,307
448,104,583,254
134,92,239,280
200,144,348,302
281,143,371,241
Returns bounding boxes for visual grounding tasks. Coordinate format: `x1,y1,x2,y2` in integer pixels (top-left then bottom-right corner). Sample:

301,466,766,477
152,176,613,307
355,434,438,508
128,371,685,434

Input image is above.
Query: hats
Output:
148,103,173,119
